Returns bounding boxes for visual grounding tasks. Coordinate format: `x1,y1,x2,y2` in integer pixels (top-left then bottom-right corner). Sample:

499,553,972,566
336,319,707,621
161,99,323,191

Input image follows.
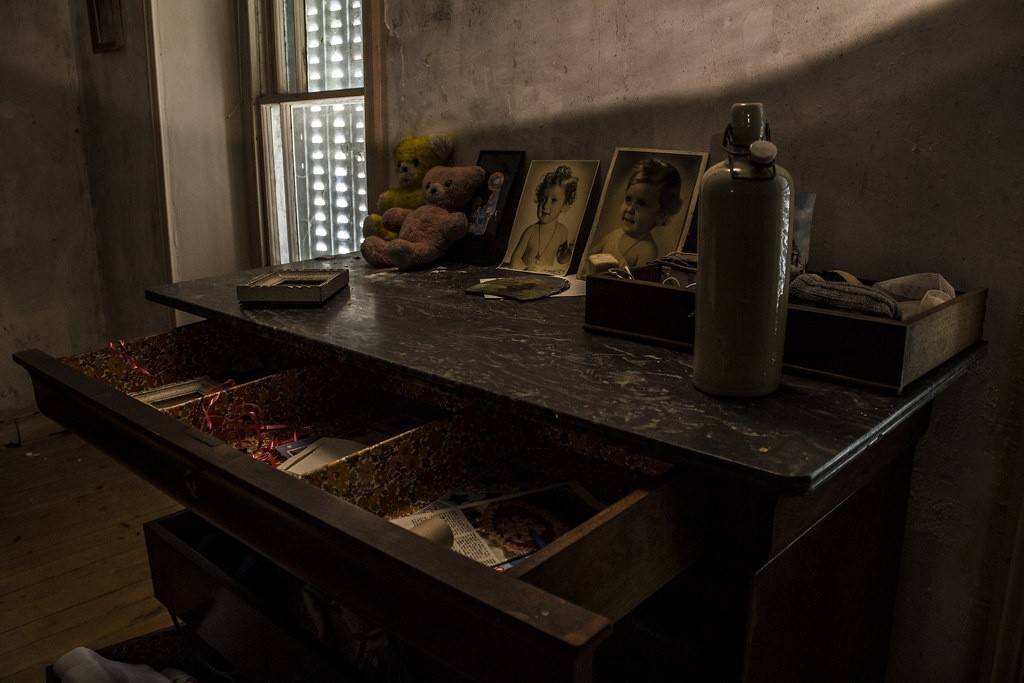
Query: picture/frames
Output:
86,0,124,55
576,145,710,281
459,149,525,267
237,267,350,304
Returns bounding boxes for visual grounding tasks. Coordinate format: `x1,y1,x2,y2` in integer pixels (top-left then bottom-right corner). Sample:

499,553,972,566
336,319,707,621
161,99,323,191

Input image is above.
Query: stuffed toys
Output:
362,134,453,241
360,166,485,270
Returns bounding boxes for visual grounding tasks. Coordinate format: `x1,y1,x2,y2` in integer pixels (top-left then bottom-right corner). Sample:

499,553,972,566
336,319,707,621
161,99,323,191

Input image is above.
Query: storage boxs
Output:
582,266,989,397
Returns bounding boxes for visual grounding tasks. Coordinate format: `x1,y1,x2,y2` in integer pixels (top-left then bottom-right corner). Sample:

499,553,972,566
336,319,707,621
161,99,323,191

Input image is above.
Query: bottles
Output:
693,101,793,397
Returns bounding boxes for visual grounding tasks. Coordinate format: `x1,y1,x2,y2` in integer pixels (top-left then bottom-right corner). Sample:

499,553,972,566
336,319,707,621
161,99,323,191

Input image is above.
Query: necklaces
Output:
618,233,650,262
535,222,558,264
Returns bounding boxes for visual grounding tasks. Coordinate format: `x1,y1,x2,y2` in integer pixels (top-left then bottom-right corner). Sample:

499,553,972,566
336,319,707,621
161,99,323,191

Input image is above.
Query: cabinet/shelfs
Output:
11,250,990,683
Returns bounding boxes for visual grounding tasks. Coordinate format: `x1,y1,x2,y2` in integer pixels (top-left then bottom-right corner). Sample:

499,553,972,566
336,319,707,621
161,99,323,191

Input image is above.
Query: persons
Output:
511,166,576,271
580,159,683,280
